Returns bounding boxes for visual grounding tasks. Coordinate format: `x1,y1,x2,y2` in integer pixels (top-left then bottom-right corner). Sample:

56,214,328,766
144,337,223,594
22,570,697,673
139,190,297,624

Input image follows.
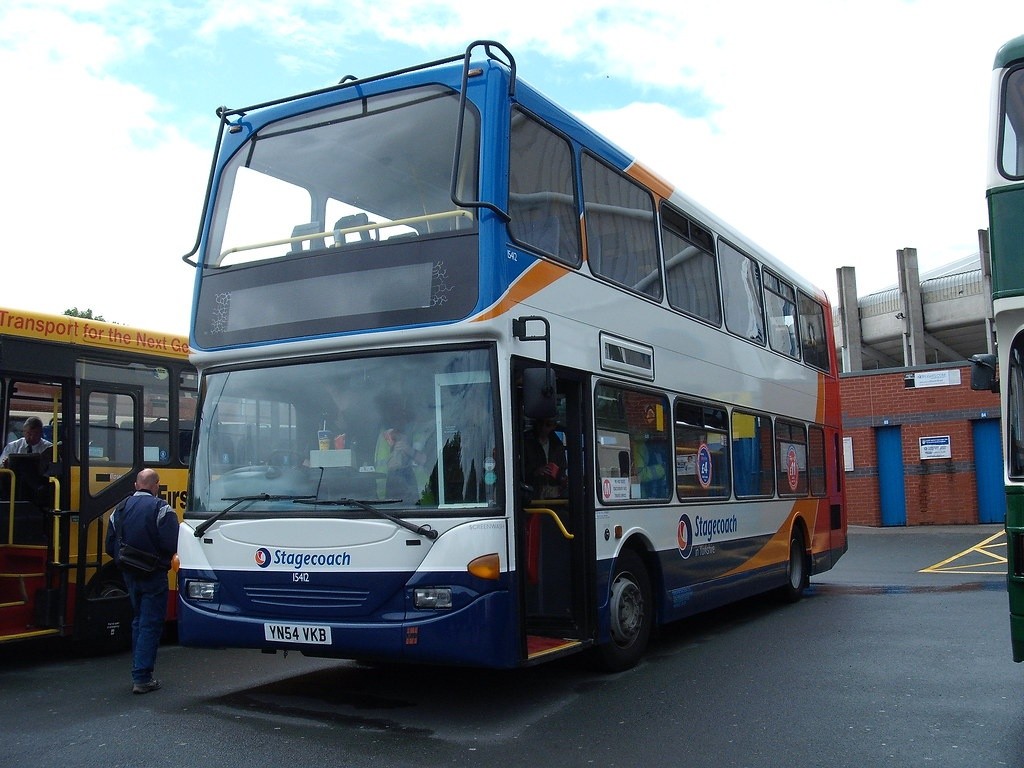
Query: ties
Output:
27,445,33,454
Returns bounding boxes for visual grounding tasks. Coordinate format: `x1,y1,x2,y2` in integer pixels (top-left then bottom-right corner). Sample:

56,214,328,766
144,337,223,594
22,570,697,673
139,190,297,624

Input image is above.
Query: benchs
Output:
148,417,169,450
48,416,119,461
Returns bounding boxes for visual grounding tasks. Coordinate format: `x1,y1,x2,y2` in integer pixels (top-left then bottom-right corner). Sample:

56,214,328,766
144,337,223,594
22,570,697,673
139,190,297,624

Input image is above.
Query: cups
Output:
318,430,331,450
334,431,346,449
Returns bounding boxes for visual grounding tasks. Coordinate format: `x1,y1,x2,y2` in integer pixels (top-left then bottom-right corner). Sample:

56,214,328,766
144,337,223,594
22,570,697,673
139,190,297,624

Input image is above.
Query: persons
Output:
0,417,53,467
105,468,179,694
303,382,666,507
685,456,698,474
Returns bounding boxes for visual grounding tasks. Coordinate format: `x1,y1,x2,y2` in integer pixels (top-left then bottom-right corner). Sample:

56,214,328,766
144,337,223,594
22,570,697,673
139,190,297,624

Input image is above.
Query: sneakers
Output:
133,679,163,694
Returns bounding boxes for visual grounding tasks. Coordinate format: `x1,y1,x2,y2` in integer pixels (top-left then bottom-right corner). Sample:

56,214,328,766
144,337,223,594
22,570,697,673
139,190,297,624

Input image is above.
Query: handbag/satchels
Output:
114,496,163,579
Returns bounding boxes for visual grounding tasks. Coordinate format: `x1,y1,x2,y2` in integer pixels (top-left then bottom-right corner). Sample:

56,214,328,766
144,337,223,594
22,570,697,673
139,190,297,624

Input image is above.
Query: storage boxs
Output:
310,449,358,470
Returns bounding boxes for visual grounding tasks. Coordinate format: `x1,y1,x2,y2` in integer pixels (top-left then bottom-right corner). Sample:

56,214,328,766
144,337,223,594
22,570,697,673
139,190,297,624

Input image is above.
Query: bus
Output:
173,35,862,684
974,31,1024,665
1,304,312,646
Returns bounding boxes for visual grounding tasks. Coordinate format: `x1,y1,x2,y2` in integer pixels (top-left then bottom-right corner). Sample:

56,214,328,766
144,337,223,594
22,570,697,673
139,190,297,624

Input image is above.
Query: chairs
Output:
286,221,328,256
333,213,379,246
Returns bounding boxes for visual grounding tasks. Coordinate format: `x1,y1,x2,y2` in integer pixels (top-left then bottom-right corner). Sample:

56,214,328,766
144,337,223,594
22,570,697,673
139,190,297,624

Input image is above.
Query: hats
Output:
538,407,561,421
